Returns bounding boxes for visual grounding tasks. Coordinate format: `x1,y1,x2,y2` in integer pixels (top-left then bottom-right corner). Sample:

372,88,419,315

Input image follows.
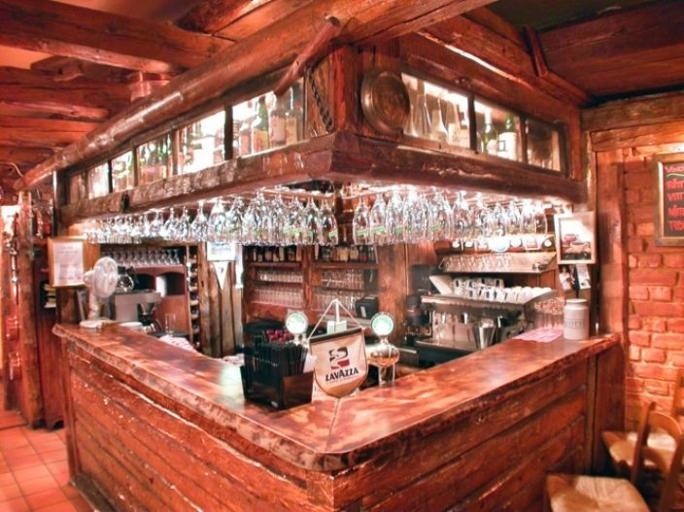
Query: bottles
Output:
133,82,305,183
399,74,523,162
562,298,592,341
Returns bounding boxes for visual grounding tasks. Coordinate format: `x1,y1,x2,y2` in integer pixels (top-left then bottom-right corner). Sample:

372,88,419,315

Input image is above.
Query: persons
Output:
557,266,574,291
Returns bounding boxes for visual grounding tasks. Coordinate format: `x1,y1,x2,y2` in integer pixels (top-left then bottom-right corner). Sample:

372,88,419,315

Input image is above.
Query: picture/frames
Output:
553,210,597,265
46,236,88,289
653,152,684,247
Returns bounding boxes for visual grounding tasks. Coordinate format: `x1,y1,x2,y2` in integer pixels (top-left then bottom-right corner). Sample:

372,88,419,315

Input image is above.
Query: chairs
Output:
545,400,684,512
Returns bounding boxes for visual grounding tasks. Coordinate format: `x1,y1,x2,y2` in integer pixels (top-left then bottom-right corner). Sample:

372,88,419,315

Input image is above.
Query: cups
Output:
564,233,590,255
448,276,556,304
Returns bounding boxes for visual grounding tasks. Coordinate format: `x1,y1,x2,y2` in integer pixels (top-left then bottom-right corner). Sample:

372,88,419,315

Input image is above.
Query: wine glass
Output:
69,181,553,272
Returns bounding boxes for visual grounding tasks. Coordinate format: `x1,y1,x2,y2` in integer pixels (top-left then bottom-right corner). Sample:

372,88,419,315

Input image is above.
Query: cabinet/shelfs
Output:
242,232,563,346
98,237,202,358
